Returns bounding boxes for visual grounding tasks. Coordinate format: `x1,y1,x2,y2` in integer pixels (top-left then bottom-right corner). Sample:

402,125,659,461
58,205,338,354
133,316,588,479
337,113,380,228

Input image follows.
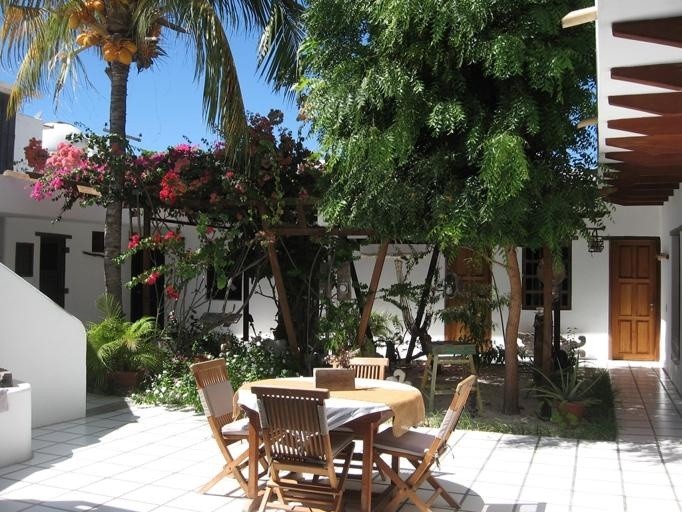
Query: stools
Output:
420,341,482,414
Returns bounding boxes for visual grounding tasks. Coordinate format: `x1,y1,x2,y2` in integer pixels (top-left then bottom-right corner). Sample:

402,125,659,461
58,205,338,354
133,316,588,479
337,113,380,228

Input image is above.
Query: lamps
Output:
586,226,606,253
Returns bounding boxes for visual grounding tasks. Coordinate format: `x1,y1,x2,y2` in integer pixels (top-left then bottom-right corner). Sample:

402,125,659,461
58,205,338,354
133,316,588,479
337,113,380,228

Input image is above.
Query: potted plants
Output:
520,350,605,421
85,293,163,394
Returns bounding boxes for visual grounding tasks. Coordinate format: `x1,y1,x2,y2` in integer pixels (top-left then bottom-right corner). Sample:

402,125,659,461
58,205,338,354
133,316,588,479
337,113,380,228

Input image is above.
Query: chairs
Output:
367,373,476,512
189,357,269,497
314,356,392,480
250,386,353,511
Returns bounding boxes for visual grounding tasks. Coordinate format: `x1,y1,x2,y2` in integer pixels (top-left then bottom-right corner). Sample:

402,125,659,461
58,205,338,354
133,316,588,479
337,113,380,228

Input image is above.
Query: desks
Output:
232,374,426,512
